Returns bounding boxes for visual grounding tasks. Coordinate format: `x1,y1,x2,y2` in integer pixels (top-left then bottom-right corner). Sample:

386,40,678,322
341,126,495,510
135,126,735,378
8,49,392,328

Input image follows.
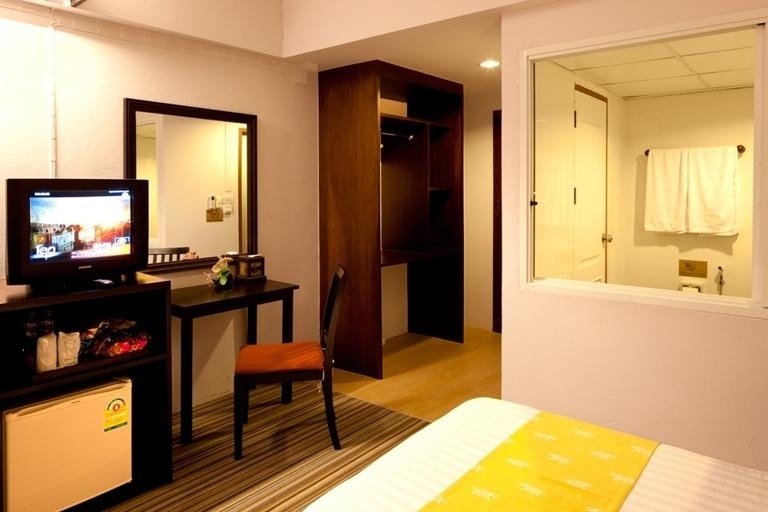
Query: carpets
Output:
111,383,432,512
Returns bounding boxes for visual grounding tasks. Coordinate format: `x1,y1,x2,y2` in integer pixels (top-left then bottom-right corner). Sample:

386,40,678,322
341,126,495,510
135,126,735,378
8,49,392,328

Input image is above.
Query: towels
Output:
685,145,738,236
645,148,687,235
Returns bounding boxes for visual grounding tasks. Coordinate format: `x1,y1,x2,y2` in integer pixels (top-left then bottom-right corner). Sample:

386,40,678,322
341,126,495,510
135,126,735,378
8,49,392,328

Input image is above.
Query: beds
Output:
299,397,768,512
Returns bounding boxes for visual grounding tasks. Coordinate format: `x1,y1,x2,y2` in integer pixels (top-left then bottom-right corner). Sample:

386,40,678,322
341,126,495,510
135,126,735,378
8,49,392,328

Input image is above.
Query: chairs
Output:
148,247,189,265
234,266,348,460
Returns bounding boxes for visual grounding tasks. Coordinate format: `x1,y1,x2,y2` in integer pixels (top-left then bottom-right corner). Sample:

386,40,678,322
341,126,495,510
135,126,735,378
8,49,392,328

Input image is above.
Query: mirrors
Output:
123,97,258,275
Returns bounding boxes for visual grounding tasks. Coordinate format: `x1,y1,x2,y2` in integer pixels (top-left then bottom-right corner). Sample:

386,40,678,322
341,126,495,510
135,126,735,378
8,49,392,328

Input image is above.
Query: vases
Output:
212,274,233,289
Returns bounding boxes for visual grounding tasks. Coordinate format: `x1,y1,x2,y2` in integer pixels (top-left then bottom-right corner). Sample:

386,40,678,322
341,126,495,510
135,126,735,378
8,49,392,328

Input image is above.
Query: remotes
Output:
93,279,113,284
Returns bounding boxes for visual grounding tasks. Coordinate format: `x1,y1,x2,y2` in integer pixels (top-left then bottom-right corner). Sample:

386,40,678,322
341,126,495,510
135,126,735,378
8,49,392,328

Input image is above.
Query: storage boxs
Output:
2,377,131,512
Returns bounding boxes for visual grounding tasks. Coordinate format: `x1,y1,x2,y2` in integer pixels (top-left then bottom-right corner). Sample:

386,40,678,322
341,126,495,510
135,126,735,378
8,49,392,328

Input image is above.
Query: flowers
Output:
182,251,199,260
202,257,235,287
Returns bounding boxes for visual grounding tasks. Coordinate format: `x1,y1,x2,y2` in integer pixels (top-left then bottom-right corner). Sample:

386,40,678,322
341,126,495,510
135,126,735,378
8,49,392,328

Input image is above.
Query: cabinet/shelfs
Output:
319,58,465,379
0,272,172,512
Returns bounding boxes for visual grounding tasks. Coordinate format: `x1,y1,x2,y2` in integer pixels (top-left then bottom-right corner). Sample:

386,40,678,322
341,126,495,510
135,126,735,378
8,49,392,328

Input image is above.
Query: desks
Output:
170,280,300,444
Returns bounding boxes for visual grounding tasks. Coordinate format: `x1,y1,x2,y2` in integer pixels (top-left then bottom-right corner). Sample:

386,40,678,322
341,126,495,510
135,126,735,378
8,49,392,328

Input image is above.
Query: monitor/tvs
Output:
6,179,149,292
